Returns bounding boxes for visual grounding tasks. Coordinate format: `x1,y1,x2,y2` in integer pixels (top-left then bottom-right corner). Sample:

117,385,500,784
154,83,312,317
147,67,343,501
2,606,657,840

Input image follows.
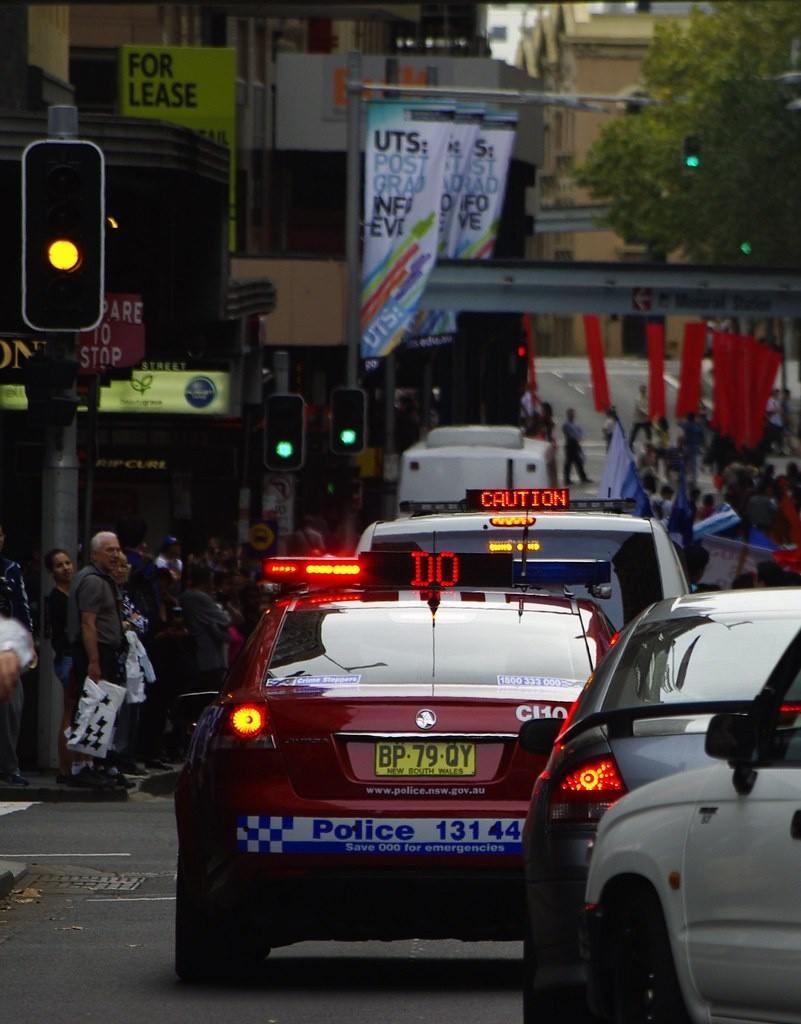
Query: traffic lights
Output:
683,136,702,170
329,388,366,456
21,138,106,333
263,393,305,471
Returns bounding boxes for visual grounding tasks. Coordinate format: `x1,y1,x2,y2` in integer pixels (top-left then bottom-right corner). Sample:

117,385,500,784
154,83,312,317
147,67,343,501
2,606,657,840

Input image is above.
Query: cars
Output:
170,549,625,991
580,623,801,1023
517,587,801,1024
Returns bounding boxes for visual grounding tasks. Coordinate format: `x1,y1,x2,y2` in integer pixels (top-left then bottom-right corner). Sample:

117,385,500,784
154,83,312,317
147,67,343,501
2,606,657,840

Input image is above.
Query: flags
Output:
666,474,693,549
597,425,656,517
362,98,519,360
694,502,740,542
522,313,537,409
711,331,783,452
646,323,665,420
675,323,708,418
583,314,612,413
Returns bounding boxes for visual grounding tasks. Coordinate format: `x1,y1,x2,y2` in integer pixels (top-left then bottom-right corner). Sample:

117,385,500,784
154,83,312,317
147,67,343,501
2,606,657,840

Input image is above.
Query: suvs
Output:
351,505,711,638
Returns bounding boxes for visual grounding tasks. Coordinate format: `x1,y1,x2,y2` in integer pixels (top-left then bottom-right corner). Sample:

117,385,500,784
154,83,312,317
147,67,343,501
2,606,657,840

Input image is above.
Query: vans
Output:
396,425,568,519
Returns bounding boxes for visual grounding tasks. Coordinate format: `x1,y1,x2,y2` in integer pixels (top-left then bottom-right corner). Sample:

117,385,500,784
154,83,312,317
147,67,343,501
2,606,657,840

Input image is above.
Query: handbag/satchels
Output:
125,629,156,703
66,676,128,758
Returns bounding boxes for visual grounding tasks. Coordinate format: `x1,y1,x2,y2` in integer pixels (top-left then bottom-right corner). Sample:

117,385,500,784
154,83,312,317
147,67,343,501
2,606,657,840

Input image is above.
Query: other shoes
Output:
2,773,28,786
104,770,135,789
73,766,111,787
124,763,149,774
146,760,172,770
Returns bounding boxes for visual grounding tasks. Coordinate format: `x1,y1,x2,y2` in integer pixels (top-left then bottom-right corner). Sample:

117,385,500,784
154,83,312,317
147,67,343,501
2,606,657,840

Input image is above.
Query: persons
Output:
0,392,439,791
519,386,796,592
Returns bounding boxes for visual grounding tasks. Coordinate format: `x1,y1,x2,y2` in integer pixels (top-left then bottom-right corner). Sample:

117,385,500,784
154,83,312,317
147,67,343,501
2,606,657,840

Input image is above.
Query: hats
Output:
162,536,180,545
